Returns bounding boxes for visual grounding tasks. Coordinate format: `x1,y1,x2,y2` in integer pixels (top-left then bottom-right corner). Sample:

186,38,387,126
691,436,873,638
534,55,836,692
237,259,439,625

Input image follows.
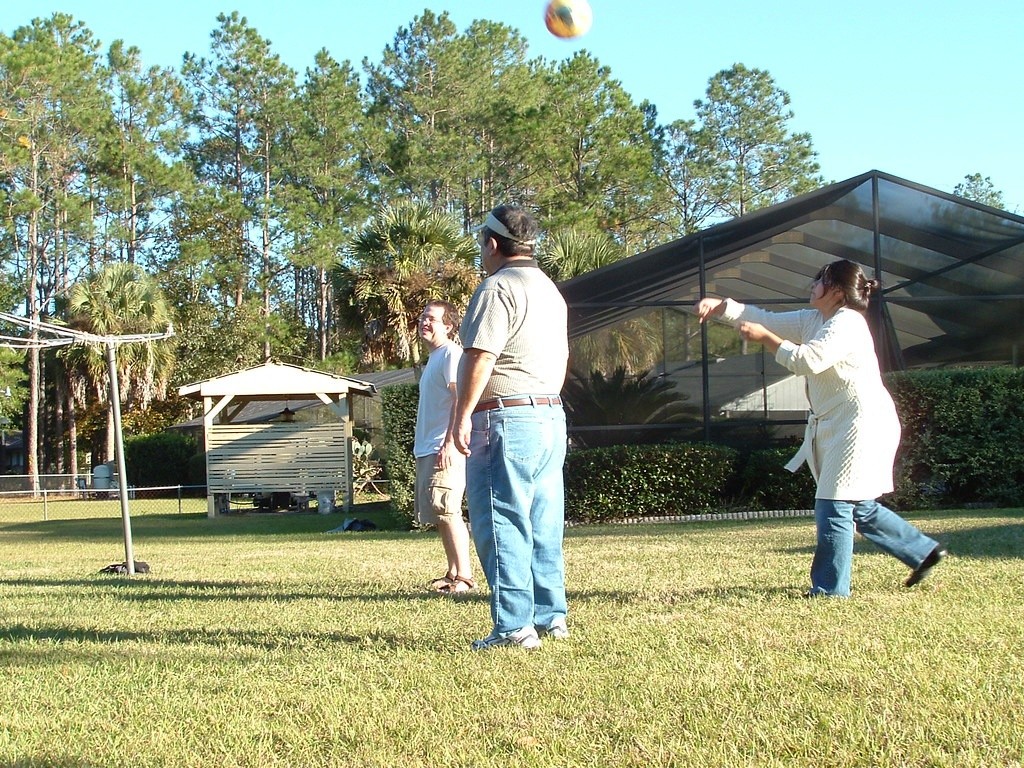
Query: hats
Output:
470,213,536,245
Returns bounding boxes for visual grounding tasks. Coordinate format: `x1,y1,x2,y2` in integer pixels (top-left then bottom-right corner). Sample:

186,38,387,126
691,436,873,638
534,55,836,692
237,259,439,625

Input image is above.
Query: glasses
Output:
418,316,444,324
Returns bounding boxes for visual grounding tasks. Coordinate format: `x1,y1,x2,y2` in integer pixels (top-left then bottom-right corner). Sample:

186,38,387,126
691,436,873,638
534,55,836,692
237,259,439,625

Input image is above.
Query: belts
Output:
472,398,560,415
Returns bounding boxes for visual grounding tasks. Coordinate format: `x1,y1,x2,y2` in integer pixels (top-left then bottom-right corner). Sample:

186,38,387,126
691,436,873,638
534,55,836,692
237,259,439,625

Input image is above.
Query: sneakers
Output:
471,624,540,651
534,619,568,639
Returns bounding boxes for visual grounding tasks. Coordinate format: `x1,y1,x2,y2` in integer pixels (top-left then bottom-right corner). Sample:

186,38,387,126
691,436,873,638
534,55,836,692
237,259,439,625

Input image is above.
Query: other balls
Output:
544,0,593,38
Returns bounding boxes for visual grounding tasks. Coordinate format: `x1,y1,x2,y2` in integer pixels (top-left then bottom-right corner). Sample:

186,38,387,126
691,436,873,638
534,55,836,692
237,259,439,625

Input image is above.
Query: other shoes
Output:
905,545,947,586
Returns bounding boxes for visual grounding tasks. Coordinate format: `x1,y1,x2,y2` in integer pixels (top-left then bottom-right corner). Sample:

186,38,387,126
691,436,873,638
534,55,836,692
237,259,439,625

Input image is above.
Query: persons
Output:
694,259,949,598
452,203,573,651
412,299,475,594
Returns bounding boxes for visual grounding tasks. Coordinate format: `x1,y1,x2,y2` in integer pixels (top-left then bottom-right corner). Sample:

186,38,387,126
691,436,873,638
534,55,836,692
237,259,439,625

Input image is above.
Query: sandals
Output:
426,572,455,590
439,575,478,593
